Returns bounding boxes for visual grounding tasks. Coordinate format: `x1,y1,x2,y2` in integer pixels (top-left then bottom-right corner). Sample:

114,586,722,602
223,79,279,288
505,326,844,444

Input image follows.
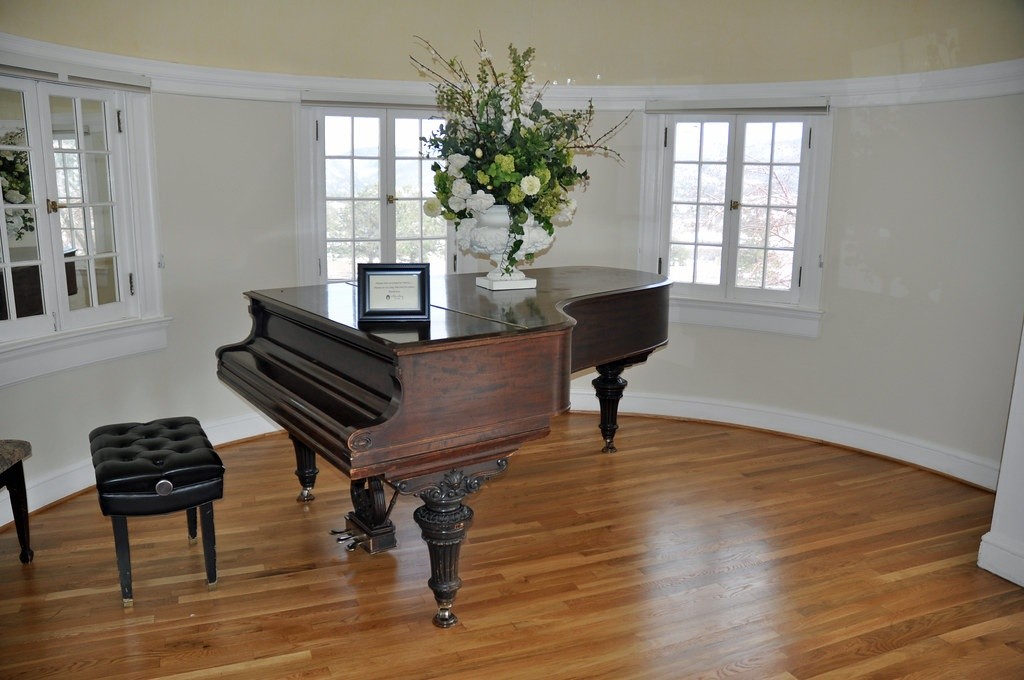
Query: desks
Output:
0,246,78,320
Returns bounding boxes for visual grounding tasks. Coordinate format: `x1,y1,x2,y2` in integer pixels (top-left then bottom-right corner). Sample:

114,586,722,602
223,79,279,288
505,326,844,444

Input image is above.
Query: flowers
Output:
408,28,635,279
0,126,35,241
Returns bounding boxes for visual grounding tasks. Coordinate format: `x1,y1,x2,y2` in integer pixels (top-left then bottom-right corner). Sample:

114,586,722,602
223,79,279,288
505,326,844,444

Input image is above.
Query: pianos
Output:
213,263,678,629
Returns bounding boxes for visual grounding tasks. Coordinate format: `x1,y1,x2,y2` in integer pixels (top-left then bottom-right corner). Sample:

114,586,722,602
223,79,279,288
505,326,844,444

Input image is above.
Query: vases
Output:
469,205,548,291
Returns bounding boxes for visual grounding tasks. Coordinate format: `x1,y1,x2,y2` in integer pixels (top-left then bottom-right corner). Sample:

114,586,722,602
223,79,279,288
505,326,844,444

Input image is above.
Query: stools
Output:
89,416,225,608
0,440,34,566
